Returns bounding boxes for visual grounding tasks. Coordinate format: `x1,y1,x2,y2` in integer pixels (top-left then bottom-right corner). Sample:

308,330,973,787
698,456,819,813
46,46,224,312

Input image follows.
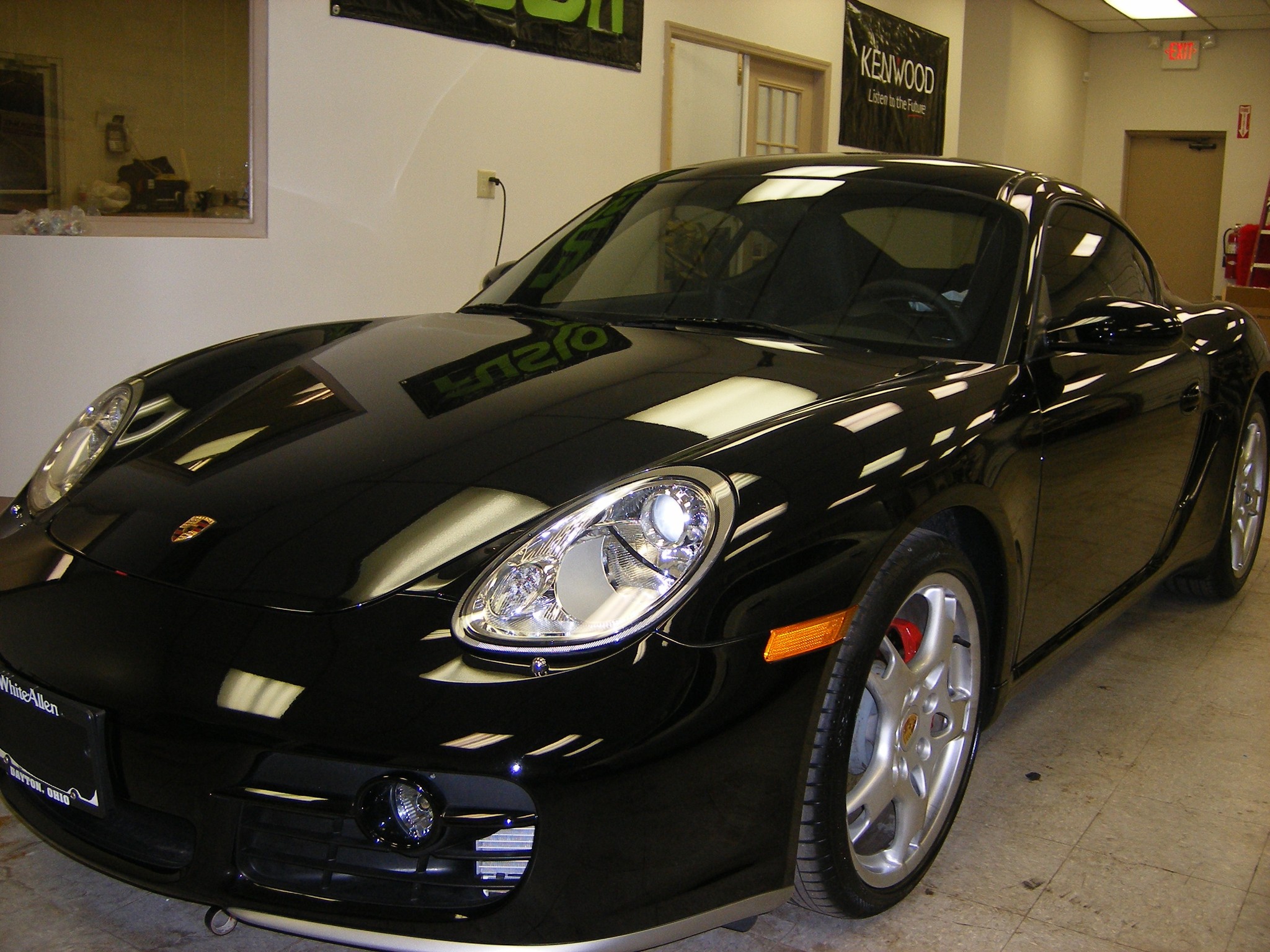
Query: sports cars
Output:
1,152,1270,952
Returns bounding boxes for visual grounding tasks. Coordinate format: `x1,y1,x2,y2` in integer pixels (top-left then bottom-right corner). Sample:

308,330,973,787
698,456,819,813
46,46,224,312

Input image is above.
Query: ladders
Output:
1246,178,1270,288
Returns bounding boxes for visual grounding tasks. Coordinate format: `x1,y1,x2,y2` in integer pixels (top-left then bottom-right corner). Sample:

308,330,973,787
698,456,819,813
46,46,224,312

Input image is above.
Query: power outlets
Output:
477,169,496,199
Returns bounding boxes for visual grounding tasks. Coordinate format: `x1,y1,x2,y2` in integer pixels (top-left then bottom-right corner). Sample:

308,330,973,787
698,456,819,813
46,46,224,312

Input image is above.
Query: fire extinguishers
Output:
1222,223,1241,279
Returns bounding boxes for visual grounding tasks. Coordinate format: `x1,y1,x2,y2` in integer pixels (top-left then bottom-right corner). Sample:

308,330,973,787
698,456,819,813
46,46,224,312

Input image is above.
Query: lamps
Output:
1148,34,1215,70
